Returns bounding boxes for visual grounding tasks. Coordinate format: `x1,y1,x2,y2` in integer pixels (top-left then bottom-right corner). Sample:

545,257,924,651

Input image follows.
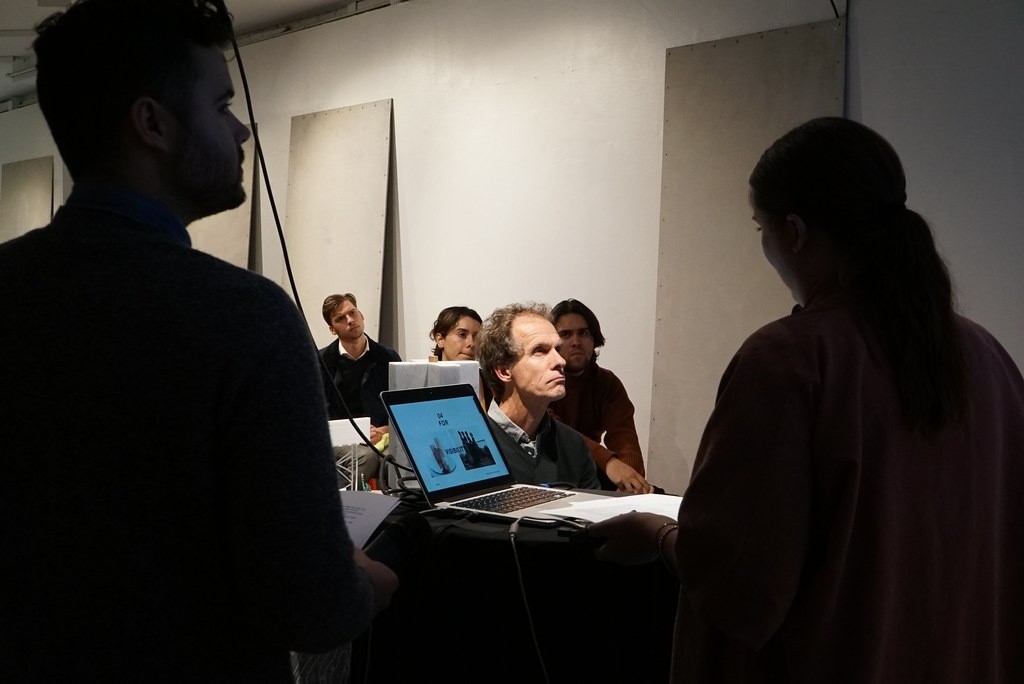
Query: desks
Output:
362,490,681,684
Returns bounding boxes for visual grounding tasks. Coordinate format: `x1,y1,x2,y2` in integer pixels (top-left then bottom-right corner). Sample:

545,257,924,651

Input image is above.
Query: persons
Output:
590,116,1024,684
0,0,400,684
429,307,483,361
547,298,655,495
315,293,402,490
476,302,601,491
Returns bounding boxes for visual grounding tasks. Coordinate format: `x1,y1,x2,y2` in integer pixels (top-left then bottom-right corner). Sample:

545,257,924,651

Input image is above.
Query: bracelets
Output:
655,522,679,546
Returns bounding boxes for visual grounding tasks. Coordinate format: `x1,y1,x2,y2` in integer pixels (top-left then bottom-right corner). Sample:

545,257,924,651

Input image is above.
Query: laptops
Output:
379,383,616,526
